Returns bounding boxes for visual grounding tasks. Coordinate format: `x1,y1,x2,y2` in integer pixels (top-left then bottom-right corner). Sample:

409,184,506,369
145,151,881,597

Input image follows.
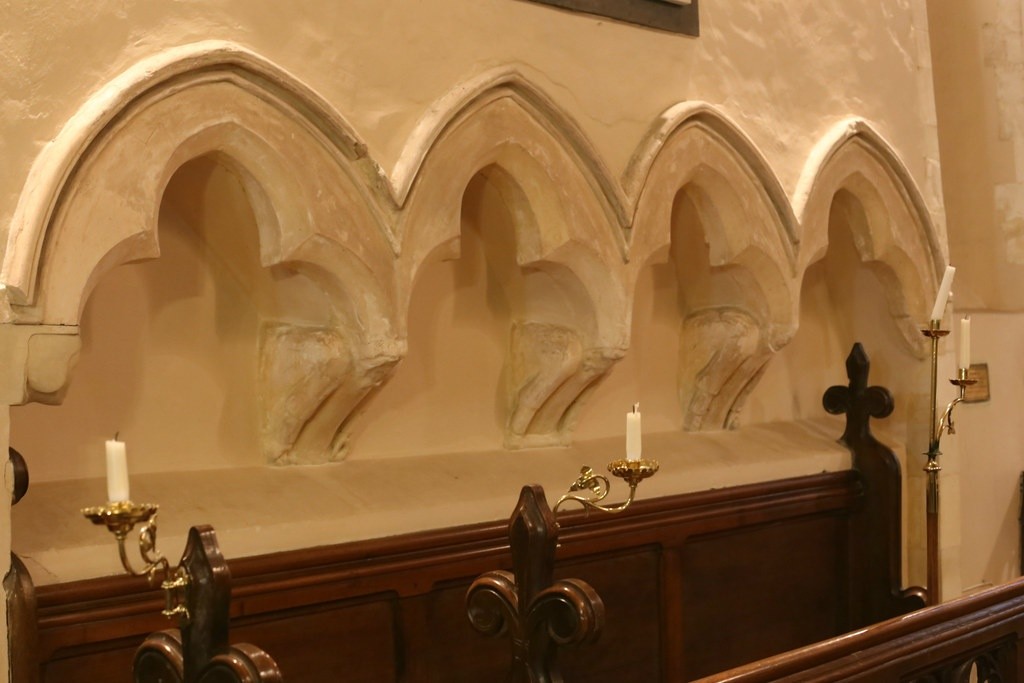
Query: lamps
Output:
105,432,130,503
626,403,642,459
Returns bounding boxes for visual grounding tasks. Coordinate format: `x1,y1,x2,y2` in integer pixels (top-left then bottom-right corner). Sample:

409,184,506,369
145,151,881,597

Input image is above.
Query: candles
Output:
960,314,972,368
932,265,955,319
